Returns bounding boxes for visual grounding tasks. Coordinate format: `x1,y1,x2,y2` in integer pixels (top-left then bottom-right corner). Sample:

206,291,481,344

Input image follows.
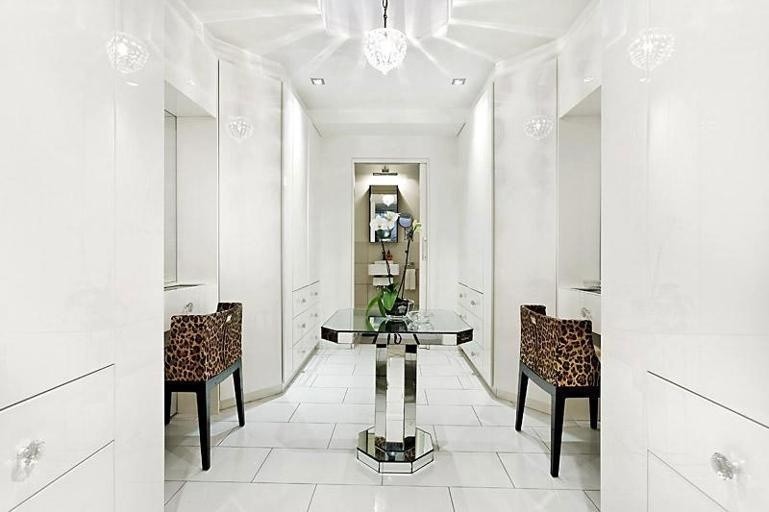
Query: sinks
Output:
571,287,601,295
374,260,393,264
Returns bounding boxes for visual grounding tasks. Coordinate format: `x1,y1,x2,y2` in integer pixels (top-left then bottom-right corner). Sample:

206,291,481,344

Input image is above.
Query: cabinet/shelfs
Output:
454,82,495,389
0,0,118,512
283,81,322,392
646,0,769,512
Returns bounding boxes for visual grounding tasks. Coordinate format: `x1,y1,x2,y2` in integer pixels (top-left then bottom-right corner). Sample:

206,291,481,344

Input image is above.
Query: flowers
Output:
364,210,423,331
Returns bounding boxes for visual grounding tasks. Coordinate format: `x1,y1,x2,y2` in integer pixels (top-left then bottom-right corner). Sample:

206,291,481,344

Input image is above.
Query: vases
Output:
384,300,408,316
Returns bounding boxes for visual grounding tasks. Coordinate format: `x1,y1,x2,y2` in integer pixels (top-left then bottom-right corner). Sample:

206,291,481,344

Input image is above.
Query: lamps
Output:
363,0,407,73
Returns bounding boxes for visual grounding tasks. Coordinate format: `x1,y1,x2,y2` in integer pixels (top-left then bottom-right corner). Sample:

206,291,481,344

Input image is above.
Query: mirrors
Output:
368,183,399,244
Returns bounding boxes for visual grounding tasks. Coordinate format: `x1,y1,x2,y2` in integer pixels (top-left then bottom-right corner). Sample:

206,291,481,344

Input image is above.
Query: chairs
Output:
164,302,246,471
514,303,601,477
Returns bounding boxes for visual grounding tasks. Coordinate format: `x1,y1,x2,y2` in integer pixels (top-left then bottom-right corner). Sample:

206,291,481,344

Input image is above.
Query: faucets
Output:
382,251,385,259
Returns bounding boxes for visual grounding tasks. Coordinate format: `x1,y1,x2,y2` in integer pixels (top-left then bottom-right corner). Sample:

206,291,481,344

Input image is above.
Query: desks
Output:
321,308,472,473
